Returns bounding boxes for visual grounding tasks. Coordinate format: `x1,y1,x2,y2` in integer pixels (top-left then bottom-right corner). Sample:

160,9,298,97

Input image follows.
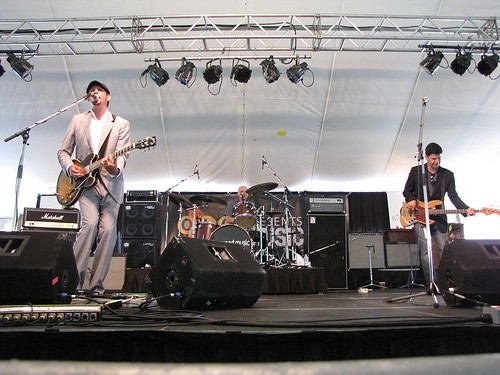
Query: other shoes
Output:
91,286,105,296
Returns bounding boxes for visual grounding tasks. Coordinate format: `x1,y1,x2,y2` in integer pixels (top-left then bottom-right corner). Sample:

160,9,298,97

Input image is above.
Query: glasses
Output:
430,174,435,187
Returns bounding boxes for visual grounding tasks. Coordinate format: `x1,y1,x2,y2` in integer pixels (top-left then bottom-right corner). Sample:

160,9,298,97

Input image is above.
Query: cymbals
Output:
189,195,227,208
168,193,194,210
263,211,282,217
245,182,278,195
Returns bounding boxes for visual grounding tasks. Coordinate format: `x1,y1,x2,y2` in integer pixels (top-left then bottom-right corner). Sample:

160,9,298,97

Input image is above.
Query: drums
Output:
193,201,257,253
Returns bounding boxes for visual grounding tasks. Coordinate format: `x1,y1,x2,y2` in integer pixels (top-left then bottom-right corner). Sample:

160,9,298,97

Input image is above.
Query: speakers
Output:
434,238,500,308
0,231,80,304
122,204,161,240
143,236,267,312
121,240,161,268
308,214,347,289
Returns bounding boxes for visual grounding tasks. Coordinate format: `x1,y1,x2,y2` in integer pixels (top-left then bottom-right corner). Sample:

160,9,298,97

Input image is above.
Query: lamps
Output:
419,51,445,76
451,52,472,75
289,61,308,84
6,52,35,79
175,58,196,84
203,58,223,85
261,56,280,82
147,58,169,87
232,59,252,83
478,53,500,77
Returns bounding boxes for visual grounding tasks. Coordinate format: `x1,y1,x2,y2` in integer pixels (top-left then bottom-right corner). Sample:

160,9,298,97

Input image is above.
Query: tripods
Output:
383,103,440,309
359,246,388,290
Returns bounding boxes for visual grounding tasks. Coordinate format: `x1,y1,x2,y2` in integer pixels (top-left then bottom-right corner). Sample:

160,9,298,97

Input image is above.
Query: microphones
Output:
423,97,428,106
262,155,264,170
89,92,100,102
195,164,200,179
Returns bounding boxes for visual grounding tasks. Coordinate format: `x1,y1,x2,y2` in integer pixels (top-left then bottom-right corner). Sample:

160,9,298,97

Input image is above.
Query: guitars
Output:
56,135,156,206
399,200,500,227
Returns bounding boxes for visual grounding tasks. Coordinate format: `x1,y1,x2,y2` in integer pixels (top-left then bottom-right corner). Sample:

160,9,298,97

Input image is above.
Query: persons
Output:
224,185,270,262
57,79,131,296
402,142,476,291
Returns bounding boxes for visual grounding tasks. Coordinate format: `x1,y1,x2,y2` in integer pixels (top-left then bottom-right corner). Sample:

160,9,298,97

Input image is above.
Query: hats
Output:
85,80,110,94
